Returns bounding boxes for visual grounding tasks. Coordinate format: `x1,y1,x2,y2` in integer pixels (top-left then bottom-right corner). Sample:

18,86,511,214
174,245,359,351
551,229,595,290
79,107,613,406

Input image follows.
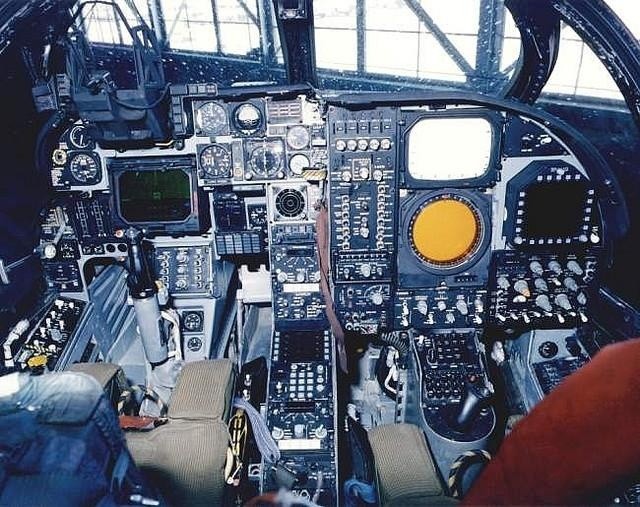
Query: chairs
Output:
366,336,640,507
0,359,241,507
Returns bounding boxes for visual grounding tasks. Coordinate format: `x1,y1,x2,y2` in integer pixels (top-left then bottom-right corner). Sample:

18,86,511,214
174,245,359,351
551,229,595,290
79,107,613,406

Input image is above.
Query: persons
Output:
455,338,640,507
